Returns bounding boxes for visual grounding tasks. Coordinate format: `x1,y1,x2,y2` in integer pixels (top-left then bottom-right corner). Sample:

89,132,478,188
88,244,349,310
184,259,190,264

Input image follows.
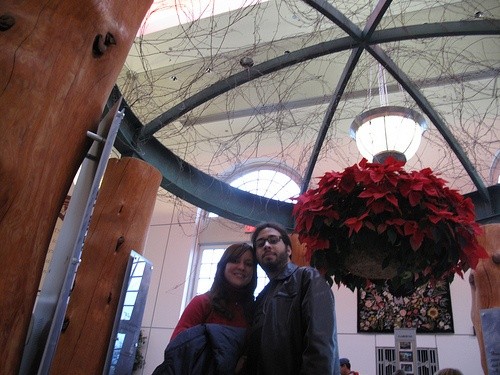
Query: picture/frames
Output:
357,282,455,335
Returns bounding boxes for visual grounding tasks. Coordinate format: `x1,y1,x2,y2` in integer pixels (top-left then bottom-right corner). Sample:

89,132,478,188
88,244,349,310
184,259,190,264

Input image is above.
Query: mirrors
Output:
103,250,154,375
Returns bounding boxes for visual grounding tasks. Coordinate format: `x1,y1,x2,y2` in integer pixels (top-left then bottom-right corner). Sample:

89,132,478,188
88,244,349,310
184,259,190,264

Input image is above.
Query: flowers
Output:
289,157,491,297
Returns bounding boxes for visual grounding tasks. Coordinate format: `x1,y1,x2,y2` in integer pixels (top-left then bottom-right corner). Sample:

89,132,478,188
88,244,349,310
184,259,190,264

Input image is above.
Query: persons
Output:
236,223,341,375
168,242,258,344
340,358,360,375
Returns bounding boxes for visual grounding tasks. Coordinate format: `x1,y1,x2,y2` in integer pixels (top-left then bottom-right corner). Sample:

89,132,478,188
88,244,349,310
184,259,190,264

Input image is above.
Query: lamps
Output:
348,62,428,164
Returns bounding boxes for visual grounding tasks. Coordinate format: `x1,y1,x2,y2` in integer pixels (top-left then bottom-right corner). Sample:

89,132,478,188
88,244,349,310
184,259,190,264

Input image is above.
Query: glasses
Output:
254,235,286,247
